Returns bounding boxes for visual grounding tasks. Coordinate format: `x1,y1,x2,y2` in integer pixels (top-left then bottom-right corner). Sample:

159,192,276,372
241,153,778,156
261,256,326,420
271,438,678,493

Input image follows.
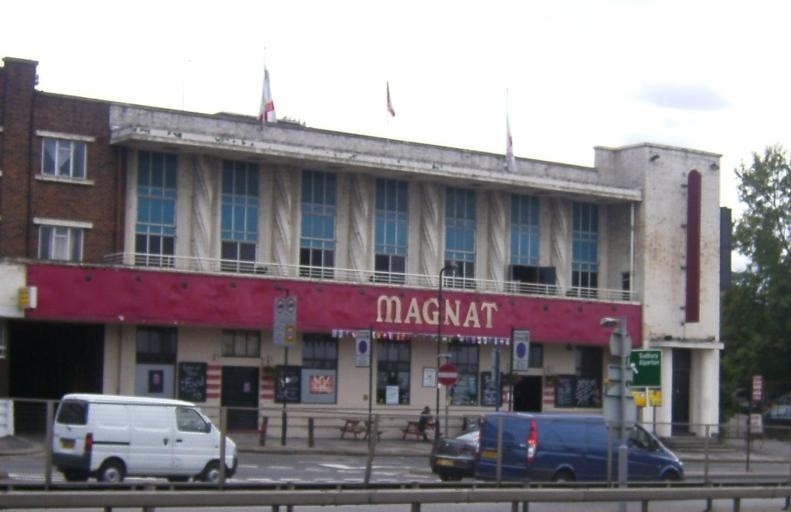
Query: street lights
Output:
272,284,291,447
599,317,629,489
445,335,460,439
432,264,459,440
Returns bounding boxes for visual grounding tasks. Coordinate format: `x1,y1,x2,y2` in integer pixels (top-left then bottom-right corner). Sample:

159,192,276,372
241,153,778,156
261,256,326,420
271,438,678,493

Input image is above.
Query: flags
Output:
386,81,396,120
258,67,277,124
506,114,518,176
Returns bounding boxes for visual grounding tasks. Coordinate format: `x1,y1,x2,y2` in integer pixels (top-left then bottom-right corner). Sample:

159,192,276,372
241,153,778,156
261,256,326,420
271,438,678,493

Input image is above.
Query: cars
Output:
428,428,481,482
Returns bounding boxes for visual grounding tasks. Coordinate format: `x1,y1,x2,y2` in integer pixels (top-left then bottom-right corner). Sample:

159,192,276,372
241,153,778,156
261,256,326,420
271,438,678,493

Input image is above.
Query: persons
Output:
423,415,437,441
419,405,443,443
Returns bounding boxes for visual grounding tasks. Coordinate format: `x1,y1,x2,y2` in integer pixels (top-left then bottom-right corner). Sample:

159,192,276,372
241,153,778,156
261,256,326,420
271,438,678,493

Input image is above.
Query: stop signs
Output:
437,363,460,388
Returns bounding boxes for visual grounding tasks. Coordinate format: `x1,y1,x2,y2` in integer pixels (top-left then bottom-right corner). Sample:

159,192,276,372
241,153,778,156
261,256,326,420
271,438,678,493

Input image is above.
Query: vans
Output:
50,391,238,485
472,412,684,488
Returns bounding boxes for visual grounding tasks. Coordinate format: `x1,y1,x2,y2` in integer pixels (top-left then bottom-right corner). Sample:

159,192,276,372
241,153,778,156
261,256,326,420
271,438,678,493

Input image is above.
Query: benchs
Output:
339,425,383,442
401,428,442,443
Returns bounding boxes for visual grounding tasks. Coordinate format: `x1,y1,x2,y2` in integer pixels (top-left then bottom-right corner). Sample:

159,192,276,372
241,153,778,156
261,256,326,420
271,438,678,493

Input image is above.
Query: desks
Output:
402,420,442,443
340,415,382,441
